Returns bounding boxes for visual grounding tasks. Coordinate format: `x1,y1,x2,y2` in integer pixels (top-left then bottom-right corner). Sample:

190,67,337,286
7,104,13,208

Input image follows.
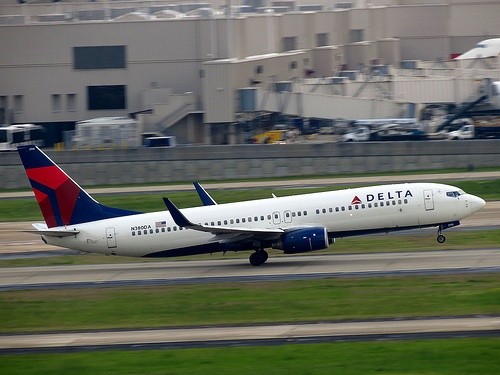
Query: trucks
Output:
343,127,426,142
71,118,176,147
448,124,500,141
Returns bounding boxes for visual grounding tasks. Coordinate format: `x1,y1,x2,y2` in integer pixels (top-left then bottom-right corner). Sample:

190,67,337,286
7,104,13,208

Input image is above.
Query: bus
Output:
0,123,47,150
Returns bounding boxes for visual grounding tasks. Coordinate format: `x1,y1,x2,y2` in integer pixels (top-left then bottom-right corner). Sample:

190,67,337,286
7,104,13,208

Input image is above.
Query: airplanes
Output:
16,144,487,266
